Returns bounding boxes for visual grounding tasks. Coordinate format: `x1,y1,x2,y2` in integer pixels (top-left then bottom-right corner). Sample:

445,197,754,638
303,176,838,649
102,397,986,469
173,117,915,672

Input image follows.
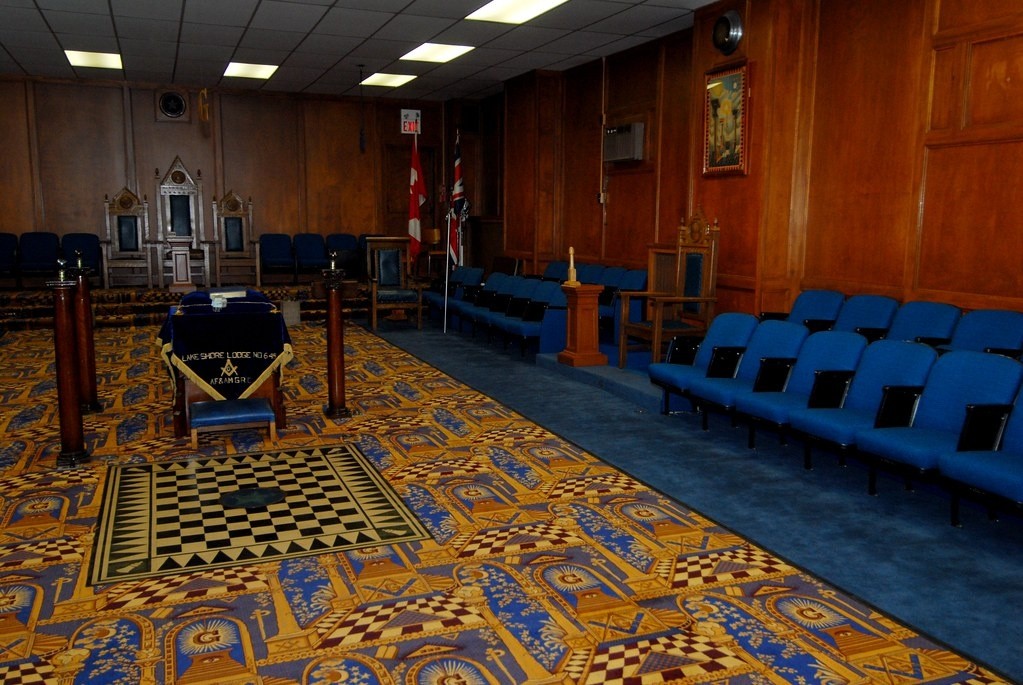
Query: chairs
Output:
212,189,260,288
259,233,386,286
366,235,421,332
616,201,721,370
103,187,153,289
420,256,649,357
0,233,103,290
647,289,1023,529
414,228,447,280
152,155,212,290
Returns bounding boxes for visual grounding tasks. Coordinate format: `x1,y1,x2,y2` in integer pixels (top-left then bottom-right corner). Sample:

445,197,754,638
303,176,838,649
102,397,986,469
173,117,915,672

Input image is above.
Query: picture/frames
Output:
703,56,749,178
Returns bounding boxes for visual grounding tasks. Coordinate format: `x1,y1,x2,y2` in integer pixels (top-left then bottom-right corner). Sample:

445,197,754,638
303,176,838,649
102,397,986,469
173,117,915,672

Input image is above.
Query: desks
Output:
156,288,294,439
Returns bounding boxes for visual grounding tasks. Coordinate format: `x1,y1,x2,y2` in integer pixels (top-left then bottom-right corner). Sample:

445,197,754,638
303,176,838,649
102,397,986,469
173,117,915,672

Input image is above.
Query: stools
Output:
188,397,276,450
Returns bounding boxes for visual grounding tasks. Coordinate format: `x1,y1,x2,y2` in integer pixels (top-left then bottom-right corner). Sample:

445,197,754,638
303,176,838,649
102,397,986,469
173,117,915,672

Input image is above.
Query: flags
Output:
445,133,470,272
408,133,427,262
